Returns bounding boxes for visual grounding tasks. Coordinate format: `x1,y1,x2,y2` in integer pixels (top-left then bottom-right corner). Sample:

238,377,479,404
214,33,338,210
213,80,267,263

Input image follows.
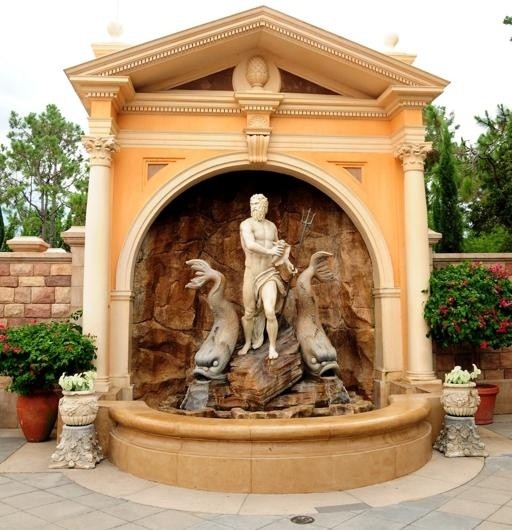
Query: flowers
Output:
445,364,481,384
58,369,97,392
420,259,512,382
0,318,98,396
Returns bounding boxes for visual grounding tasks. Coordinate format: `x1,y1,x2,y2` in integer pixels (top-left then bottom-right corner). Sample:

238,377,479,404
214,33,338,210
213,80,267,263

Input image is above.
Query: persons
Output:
237,194,286,359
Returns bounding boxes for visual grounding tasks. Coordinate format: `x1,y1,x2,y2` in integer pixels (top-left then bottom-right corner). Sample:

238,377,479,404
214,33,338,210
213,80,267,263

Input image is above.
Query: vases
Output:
440,382,481,416
16,386,59,442
58,391,99,426
474,383,499,425
